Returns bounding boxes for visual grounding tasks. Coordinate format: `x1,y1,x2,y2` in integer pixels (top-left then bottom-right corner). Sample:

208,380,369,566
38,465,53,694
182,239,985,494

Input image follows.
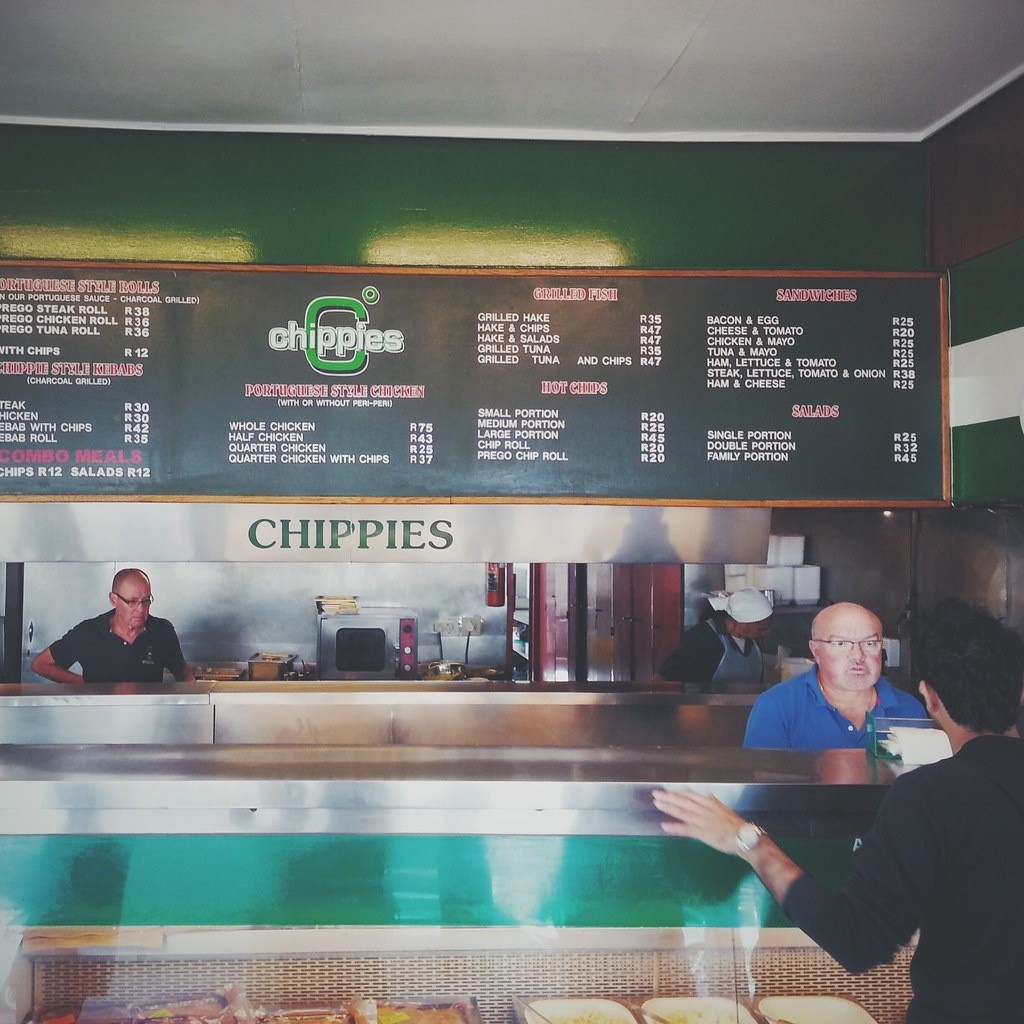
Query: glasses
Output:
816,639,883,653
116,593,154,607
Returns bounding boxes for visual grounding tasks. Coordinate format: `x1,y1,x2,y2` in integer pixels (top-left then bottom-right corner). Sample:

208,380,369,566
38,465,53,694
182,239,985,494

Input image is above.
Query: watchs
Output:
735,821,768,857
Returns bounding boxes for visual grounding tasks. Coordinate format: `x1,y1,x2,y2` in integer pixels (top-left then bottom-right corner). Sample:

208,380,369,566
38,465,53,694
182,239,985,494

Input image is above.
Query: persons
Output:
742,602,928,750
32,568,196,684
652,586,773,682
651,596,1024,1024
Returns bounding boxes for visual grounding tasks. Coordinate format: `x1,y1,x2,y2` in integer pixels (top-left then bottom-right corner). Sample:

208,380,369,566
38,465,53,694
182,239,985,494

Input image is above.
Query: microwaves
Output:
315,606,419,681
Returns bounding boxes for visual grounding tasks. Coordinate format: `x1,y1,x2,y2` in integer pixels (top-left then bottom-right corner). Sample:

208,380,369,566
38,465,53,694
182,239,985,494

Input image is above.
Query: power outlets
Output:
433,616,459,636
881,637,900,667
459,616,481,636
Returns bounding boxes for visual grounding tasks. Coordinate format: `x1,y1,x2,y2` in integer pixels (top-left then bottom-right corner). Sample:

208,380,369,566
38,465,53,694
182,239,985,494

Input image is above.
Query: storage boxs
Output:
314,595,362,616
